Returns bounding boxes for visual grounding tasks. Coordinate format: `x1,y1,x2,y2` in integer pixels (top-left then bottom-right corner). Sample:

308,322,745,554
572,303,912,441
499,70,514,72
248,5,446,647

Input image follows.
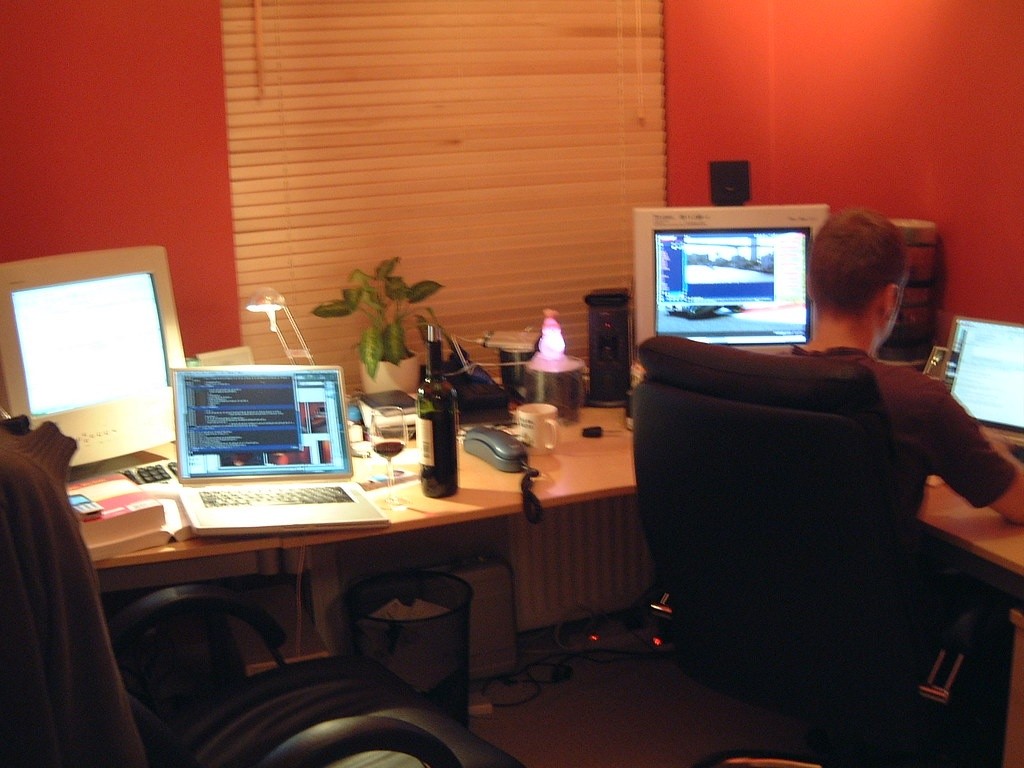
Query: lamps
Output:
245,285,315,366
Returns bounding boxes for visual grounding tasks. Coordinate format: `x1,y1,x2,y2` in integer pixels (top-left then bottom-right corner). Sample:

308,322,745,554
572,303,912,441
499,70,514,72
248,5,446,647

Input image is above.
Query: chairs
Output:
0,415,529,767
632,335,997,768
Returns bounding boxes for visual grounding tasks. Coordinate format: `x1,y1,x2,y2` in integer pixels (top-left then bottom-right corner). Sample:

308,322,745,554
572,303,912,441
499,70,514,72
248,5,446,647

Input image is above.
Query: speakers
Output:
708,160,752,205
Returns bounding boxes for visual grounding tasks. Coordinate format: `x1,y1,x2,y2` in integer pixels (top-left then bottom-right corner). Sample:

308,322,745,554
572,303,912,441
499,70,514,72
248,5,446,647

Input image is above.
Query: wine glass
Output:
371,406,408,510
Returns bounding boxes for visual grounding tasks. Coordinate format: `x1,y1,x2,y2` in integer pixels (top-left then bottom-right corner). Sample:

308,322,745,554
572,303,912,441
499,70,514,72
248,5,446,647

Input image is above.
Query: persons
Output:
771,211,1024,610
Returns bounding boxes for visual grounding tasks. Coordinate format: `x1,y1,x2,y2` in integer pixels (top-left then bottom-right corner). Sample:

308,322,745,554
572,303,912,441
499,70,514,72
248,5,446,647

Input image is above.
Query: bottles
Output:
416,325,458,498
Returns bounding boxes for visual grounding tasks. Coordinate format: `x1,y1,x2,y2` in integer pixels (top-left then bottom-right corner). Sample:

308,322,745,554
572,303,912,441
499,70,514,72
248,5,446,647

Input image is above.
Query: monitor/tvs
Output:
632,204,829,356
0,247,186,468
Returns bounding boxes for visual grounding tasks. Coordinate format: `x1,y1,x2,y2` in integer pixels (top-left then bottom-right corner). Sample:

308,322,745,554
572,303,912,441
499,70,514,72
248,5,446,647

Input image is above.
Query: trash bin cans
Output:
347,569,473,728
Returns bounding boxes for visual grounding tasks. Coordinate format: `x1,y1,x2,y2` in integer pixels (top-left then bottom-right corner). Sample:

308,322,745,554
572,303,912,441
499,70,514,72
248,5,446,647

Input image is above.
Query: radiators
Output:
507,494,654,633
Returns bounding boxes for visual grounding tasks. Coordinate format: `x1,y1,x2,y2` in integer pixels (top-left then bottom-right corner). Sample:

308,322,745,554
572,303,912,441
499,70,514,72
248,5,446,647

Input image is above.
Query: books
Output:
360,390,417,417
64,473,195,561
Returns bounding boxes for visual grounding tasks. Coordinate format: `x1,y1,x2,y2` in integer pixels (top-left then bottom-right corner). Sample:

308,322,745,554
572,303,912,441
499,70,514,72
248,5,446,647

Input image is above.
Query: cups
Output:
499,348,535,397
526,357,586,426
517,403,560,456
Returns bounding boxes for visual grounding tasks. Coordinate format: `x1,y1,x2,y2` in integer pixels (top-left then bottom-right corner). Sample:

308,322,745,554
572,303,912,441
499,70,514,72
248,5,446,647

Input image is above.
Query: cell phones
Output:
69,494,104,517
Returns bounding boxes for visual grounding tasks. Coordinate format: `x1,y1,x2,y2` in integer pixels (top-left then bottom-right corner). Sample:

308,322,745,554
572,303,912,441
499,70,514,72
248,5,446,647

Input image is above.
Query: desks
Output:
60,376,1024,768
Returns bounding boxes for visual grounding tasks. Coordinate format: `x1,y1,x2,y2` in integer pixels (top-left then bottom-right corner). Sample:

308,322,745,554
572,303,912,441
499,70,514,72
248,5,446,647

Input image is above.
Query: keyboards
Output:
120,458,179,494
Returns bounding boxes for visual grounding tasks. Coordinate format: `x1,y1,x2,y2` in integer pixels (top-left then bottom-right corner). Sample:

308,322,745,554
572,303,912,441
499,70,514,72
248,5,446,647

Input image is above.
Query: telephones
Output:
464,427,527,472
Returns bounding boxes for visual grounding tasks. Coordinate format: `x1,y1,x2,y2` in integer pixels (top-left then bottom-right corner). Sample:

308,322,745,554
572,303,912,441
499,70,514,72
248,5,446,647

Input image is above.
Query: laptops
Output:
941,315,1024,451
169,365,390,536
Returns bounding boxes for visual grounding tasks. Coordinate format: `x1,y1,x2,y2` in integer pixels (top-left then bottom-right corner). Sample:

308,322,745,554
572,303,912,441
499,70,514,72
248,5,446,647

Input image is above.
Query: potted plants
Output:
311,256,445,395
